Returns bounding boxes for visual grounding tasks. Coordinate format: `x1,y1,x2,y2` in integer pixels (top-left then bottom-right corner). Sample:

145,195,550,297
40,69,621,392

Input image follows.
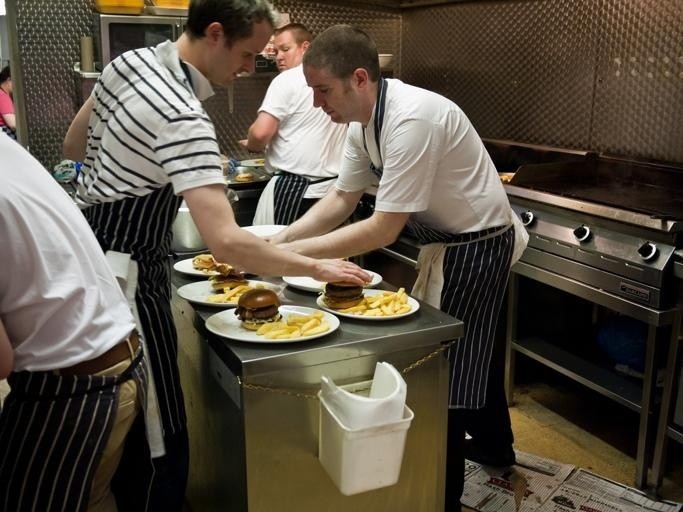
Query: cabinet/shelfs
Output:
94,14,192,75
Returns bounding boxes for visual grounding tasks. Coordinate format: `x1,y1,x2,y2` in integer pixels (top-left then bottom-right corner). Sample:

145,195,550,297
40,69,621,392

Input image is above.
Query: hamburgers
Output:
323,280,364,310
191,252,215,271
209,266,250,290
234,172,255,184
234,290,282,330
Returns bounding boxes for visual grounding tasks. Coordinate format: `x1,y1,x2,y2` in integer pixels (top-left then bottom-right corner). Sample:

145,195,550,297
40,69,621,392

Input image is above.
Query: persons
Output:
0,66,17,138
233,24,530,511
61,1,373,511
0,130,150,511
237,23,347,225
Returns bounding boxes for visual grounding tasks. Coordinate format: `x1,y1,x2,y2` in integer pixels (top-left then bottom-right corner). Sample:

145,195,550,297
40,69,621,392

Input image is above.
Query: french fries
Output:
253,312,330,340
208,284,265,304
338,287,412,318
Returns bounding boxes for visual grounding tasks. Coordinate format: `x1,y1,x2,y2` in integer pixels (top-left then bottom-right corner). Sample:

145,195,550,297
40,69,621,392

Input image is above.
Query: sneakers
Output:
465,438,515,466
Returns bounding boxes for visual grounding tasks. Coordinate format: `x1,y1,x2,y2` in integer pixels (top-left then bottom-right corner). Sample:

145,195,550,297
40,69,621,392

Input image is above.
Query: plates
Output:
279,268,384,288
202,305,340,342
173,278,284,306
316,289,422,324
173,252,243,276
237,223,291,239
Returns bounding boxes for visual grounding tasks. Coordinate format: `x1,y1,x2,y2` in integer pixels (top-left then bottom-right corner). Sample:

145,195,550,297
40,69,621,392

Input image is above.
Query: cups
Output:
80,37,95,73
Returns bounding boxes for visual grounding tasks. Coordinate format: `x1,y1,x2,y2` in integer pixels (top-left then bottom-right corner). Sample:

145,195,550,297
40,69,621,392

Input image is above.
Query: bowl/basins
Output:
165,207,207,254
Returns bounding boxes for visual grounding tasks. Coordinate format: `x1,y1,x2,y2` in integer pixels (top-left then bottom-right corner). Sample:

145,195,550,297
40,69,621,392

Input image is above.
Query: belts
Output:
59,330,139,378
